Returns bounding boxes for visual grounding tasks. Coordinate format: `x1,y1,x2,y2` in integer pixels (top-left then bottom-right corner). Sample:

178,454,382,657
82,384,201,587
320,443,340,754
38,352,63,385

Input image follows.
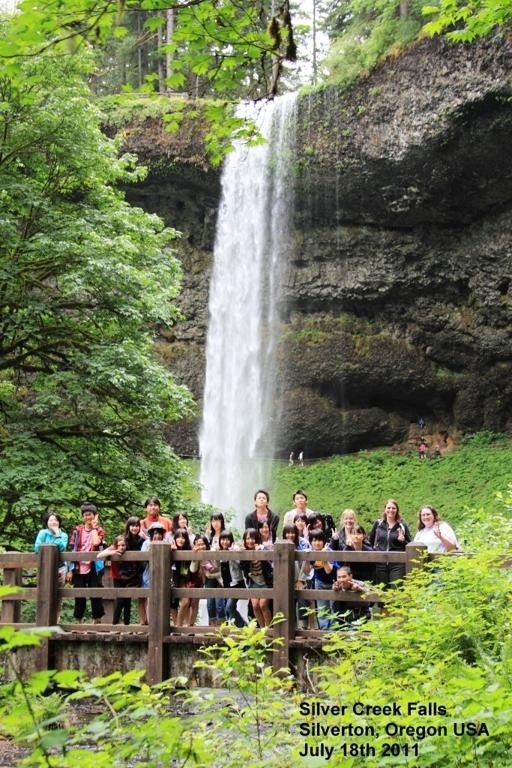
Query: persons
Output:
419,443,426,459
288,452,294,467
35,490,457,634
298,451,304,467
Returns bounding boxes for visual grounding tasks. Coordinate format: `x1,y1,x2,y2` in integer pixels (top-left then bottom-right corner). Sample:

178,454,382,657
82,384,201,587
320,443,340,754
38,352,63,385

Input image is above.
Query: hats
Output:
146,522,166,537
